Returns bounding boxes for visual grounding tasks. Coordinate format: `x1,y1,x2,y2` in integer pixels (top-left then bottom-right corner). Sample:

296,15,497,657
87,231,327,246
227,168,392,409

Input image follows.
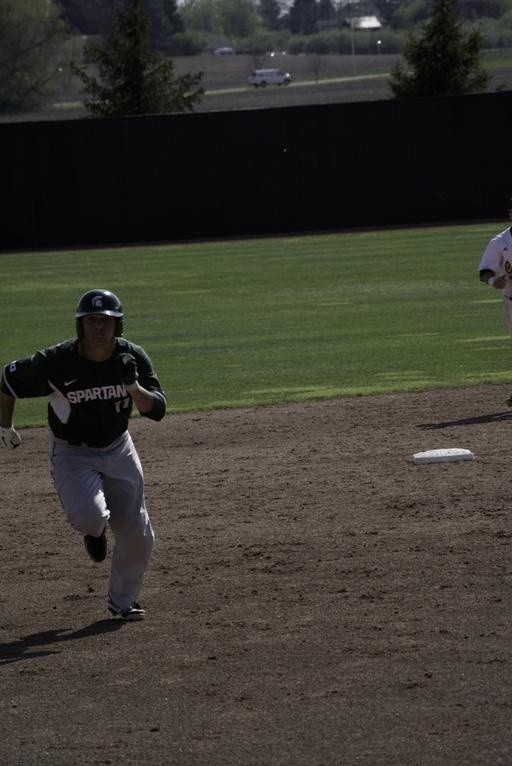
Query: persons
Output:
1,288,169,617
477,223,511,405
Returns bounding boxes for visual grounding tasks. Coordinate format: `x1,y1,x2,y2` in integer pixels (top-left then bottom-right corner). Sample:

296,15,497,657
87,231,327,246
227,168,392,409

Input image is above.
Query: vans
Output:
248,68,291,88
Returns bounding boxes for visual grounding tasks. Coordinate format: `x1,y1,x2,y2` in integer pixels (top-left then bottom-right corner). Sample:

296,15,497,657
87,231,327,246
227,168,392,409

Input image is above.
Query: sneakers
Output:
107,597,147,623
81,526,108,564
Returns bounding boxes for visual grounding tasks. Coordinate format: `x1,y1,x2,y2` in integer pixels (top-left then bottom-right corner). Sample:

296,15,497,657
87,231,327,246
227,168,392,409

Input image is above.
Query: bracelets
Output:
487,277,496,286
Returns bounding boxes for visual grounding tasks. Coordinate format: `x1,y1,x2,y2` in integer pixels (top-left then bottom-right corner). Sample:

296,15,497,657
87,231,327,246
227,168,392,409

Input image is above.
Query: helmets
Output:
74,288,124,321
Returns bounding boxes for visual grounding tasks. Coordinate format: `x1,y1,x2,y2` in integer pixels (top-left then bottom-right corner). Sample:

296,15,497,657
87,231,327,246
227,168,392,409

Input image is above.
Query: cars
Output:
214,47,254,55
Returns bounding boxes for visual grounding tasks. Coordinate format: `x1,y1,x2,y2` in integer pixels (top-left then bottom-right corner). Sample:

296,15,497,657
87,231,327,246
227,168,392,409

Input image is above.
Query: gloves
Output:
0,423,23,452
111,352,137,384
